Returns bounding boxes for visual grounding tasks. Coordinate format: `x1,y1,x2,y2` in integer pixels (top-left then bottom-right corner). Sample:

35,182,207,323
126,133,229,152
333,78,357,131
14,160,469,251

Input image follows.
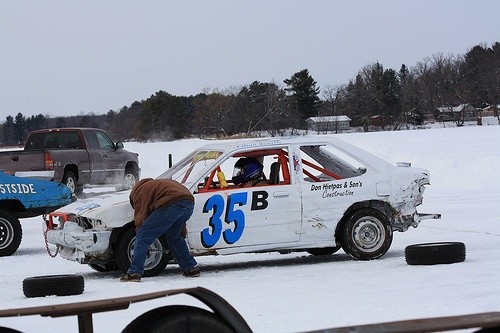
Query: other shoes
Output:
120,273,140,282
184,266,200,277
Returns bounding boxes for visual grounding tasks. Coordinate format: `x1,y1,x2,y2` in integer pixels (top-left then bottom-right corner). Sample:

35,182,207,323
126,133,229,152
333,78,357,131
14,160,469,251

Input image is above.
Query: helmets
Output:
232,157,263,184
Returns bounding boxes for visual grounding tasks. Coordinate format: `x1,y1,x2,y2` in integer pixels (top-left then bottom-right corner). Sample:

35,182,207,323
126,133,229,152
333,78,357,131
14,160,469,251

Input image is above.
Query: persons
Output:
232,156,270,186
120,178,201,283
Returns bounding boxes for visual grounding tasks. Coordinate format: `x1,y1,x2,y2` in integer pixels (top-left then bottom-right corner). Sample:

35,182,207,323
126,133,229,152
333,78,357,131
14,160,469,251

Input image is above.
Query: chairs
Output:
269,162,280,184
